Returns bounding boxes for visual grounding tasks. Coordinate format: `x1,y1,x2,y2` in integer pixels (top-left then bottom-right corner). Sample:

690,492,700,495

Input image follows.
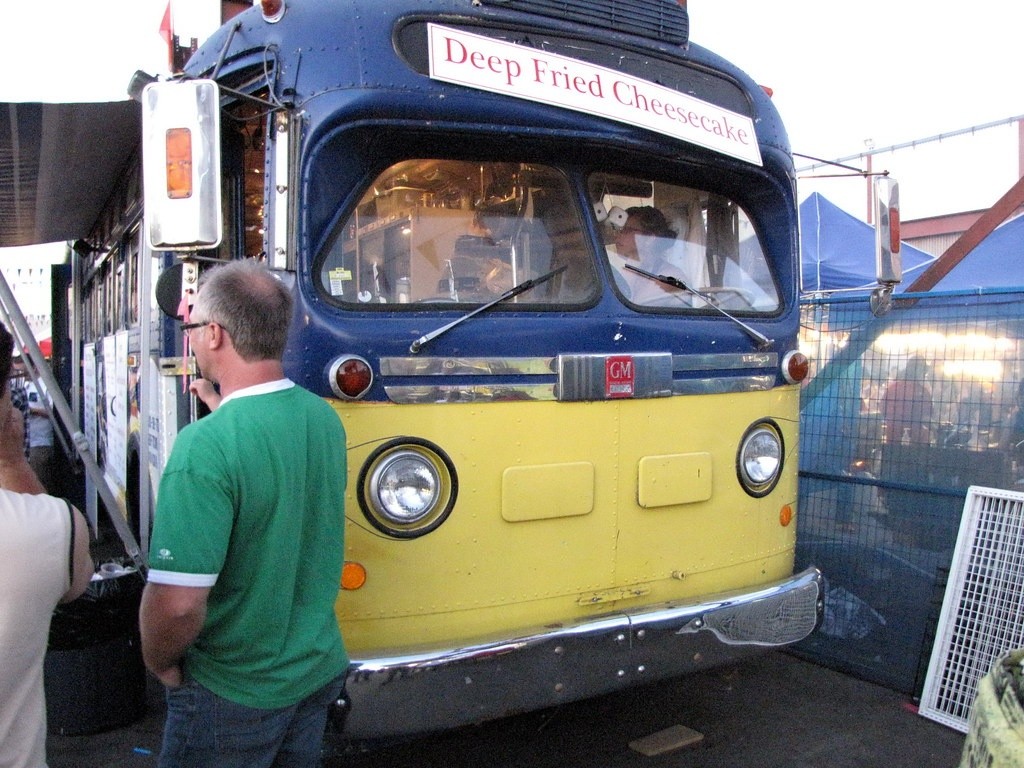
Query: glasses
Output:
180,321,226,336
621,224,652,235
6,369,29,389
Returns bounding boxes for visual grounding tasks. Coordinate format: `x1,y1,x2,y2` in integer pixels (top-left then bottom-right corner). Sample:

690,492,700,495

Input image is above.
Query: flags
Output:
159,0,173,71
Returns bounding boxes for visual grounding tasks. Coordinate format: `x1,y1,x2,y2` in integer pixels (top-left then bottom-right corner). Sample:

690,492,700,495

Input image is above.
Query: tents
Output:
738,193,942,326
930,213,1023,321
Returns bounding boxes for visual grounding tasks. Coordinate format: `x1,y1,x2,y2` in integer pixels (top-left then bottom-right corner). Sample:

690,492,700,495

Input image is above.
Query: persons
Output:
606,204,694,307
0,323,96,768
436,204,523,306
139,260,349,766
10,352,53,492
831,350,1023,486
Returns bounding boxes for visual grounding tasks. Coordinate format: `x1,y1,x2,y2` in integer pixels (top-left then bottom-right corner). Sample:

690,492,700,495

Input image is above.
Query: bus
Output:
53,1,902,747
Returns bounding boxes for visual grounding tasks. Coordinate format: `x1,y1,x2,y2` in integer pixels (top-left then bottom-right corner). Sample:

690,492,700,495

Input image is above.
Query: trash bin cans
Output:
43,558,147,734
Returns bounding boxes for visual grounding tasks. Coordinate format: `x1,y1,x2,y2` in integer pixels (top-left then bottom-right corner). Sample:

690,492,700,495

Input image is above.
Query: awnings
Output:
0,101,141,246
